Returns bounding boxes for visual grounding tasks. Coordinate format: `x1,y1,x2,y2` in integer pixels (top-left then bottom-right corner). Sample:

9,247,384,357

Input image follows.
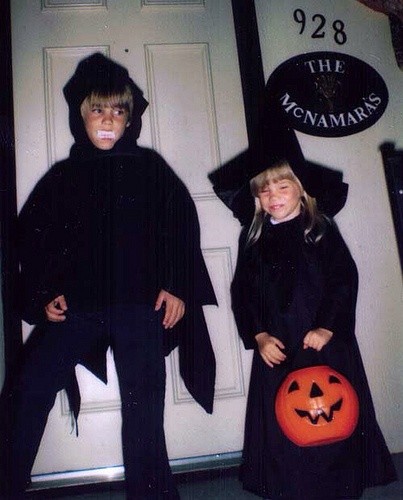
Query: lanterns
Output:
276,366,359,445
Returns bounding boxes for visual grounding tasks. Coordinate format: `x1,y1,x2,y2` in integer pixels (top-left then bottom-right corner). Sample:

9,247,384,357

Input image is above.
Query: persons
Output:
0,53,218,500
209,148,398,500
